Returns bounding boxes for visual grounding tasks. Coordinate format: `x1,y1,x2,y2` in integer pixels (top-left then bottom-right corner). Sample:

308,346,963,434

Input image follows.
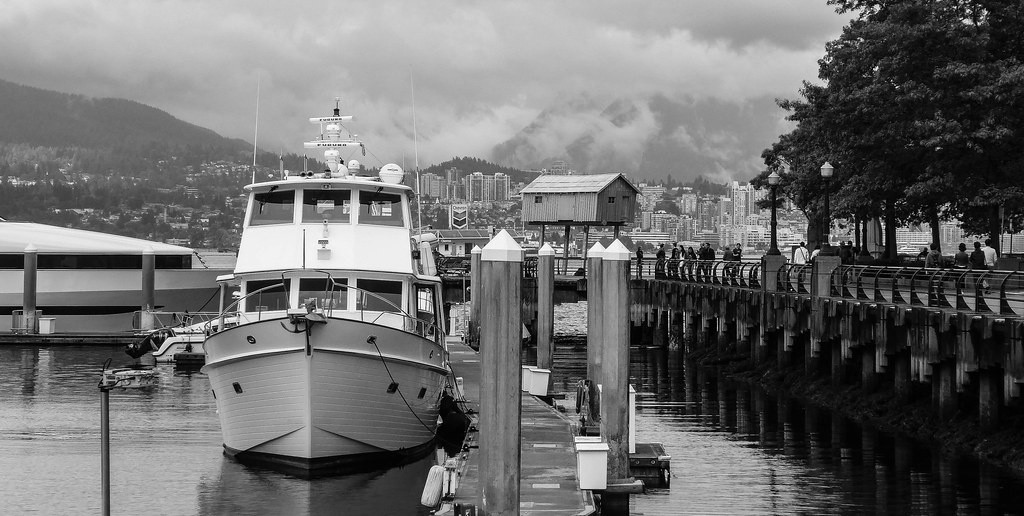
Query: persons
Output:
794,242,821,281
918,247,929,257
655,244,666,270
731,243,742,277
636,247,643,280
686,246,697,276
671,242,686,276
982,238,998,293
835,240,856,283
925,244,945,299
695,243,715,278
952,242,970,293
723,247,733,280
860,245,871,256
969,241,985,297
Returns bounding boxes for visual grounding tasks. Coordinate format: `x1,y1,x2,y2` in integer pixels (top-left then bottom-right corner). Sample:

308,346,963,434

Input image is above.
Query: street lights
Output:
819,161,834,256
766,170,782,256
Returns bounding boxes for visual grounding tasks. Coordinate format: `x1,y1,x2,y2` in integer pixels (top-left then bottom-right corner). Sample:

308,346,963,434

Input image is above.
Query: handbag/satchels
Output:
805,260,810,264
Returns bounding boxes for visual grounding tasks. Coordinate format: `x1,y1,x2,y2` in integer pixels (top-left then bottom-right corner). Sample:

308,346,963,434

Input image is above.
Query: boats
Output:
200,97,450,474
134,317,235,363
